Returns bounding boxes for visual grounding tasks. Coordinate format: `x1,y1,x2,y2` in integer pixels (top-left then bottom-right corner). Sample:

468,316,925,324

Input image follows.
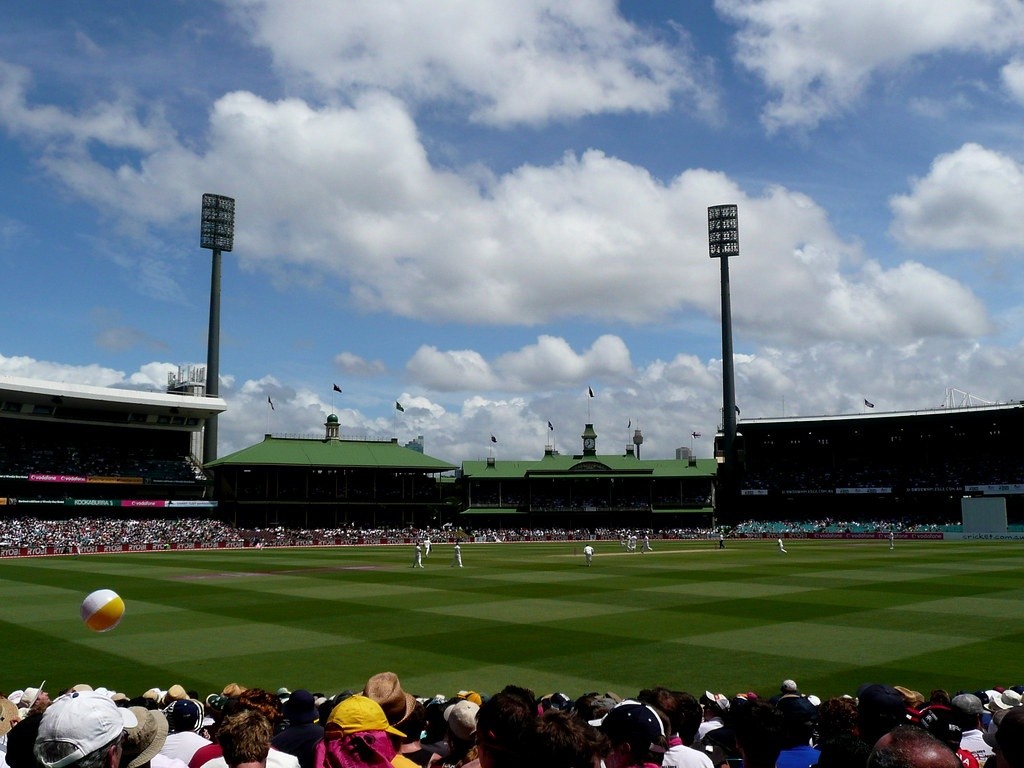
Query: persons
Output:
719,531,726,549
583,544,594,567
412,535,463,569
243,515,471,538
744,462,1024,488
737,515,960,533
0,513,242,548
720,525,740,538
472,526,700,540
777,536,787,554
0,430,196,479
729,679,1024,768
622,534,653,554
701,527,719,538
0,672,729,768
888,530,894,550
473,481,712,509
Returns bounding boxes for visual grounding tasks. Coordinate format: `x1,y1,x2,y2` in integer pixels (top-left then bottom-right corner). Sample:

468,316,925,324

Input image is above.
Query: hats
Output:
0,674,1024,768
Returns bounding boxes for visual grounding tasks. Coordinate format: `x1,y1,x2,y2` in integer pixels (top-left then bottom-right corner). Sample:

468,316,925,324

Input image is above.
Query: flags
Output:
491,434,497,443
268,397,274,410
396,402,405,412
549,421,553,430
333,383,342,392
735,404,740,415
865,399,874,408
589,388,594,397
627,419,632,428
